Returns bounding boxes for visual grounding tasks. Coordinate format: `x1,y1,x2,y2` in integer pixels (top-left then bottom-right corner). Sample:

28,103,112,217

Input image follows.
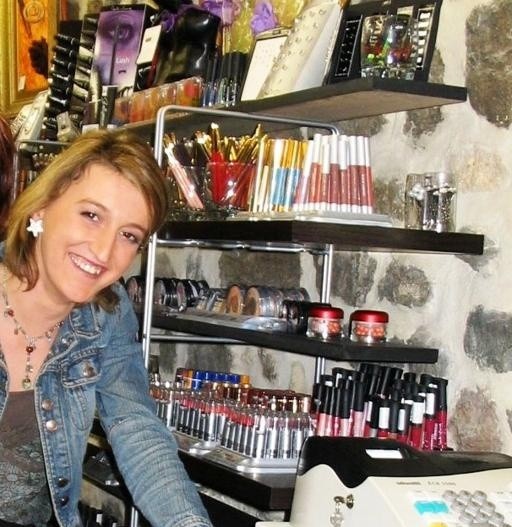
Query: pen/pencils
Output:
254,135,373,214
202,123,262,212
162,131,206,211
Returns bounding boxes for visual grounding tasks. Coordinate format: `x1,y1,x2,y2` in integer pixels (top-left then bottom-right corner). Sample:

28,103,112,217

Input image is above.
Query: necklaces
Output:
1,263,65,388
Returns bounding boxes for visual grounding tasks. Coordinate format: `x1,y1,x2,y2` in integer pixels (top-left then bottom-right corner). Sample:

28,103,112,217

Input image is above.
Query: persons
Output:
0,113,22,244
0,126,215,527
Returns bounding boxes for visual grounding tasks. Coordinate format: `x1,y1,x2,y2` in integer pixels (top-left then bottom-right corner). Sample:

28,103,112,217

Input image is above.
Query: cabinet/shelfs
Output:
8,75,512,527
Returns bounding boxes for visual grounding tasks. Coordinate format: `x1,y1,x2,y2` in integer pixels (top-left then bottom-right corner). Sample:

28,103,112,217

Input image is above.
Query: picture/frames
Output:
0,0,69,118
